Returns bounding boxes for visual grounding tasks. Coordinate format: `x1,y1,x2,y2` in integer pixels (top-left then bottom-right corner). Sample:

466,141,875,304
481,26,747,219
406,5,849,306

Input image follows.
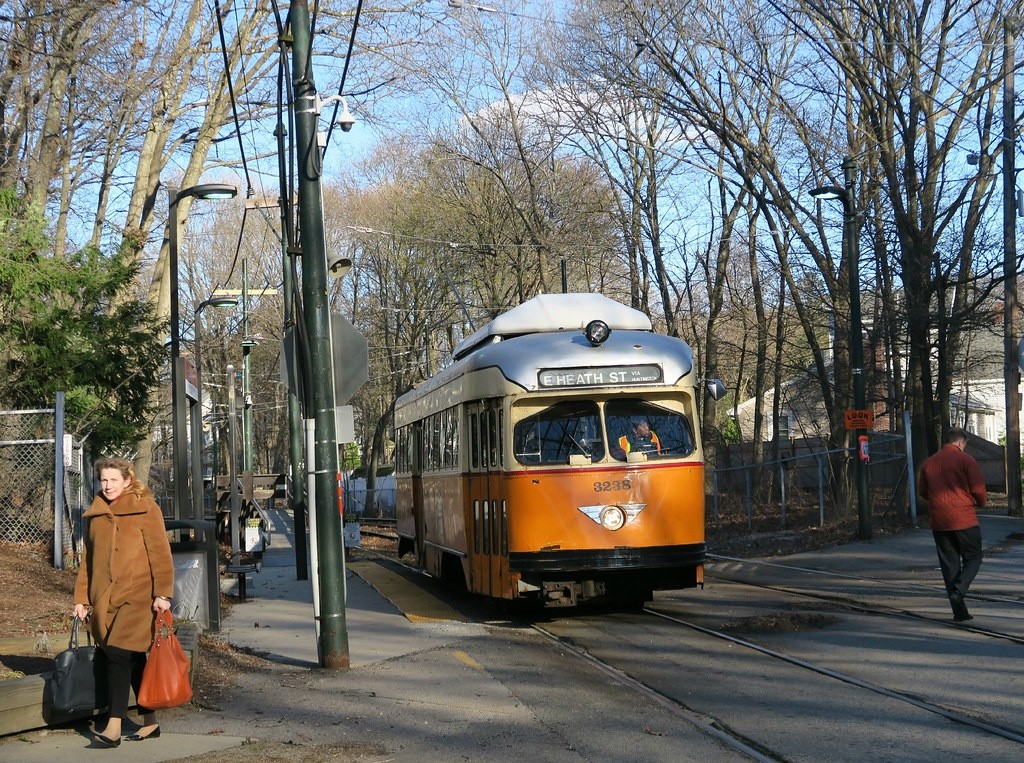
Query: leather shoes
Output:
126,725,161,741
95,735,121,748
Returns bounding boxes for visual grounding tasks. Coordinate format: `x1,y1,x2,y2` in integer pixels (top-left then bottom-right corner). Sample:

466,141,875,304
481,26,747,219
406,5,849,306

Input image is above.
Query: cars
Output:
349,463,395,480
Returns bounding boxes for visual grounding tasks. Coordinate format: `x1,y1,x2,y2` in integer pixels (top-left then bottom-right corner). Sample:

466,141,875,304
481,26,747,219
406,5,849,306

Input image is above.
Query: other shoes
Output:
954,614,974,623
951,591,965,609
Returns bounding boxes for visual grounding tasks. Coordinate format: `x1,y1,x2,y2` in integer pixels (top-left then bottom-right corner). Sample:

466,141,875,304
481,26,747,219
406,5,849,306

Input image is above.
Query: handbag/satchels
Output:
49,614,108,712
136,608,193,709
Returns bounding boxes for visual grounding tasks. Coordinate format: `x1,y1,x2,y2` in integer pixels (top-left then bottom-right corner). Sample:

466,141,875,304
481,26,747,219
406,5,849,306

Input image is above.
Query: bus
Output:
394,291,727,609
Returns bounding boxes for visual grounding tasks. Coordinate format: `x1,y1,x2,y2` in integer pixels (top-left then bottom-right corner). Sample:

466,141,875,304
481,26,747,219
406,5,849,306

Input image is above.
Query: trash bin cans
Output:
244,517,265,553
165,520,221,634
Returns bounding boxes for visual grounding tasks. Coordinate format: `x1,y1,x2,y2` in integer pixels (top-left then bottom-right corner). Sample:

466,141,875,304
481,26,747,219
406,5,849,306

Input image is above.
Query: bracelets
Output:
160,597,170,601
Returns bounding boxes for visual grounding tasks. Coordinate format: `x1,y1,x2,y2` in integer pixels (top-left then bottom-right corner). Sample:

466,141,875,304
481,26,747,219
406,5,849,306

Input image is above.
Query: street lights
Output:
810,186,874,540
283,245,351,580
240,340,259,472
194,297,237,542
169,183,238,542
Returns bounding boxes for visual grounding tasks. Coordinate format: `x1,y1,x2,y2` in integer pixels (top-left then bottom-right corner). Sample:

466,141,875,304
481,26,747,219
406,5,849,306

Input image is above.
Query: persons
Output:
618,413,661,457
918,427,988,623
72,458,174,748
